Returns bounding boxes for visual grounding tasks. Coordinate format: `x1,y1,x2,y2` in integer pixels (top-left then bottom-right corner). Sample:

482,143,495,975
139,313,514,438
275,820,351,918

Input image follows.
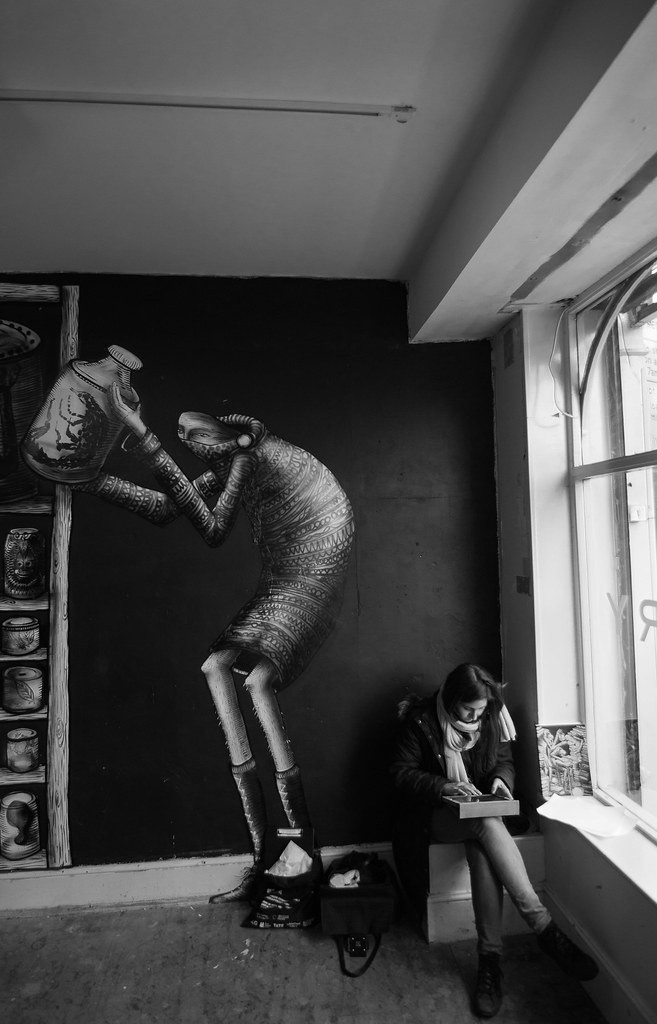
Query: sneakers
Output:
536,919,599,982
474,952,504,1017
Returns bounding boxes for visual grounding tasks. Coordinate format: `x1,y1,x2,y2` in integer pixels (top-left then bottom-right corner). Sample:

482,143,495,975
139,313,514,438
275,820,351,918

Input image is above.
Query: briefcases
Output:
317,851,395,977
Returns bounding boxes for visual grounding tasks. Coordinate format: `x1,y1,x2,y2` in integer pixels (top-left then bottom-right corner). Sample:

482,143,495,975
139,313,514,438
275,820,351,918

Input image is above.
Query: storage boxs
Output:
318,841,404,934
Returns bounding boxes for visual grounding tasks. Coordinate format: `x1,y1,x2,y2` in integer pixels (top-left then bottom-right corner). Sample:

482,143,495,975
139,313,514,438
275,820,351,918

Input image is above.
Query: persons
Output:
385,662,600,1019
